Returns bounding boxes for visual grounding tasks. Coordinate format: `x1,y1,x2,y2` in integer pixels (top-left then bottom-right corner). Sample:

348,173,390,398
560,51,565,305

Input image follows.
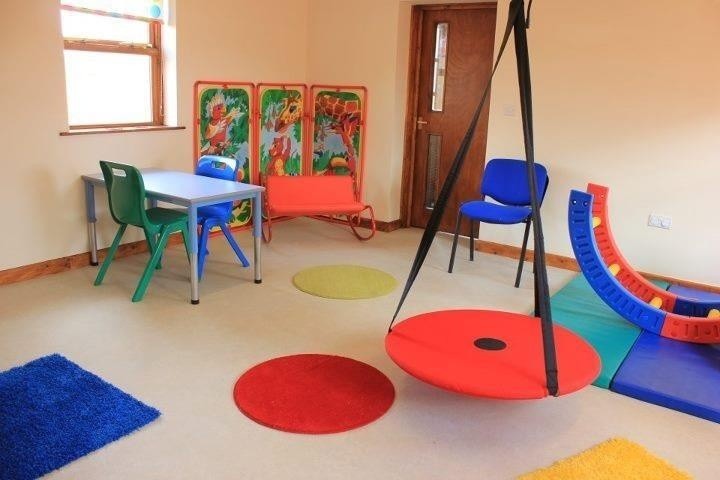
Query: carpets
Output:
512,433,693,480
0,352,164,479
292,262,401,301
234,352,399,439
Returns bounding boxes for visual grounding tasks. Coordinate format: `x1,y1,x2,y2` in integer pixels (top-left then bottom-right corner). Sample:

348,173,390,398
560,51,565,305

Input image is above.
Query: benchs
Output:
255,173,377,244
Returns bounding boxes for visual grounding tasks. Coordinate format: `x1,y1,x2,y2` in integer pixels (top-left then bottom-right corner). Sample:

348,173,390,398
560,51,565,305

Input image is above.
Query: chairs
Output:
446,156,550,289
92,153,250,303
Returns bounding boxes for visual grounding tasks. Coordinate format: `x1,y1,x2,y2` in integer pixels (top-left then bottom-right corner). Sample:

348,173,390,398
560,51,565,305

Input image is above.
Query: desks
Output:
80,165,267,306
